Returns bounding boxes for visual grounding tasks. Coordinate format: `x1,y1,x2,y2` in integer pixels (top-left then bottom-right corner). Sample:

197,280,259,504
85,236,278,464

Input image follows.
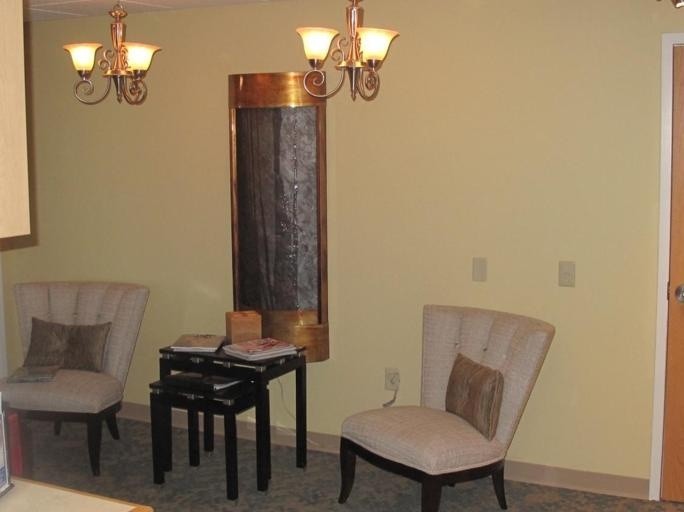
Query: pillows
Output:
446,353,503,441
23,316,112,375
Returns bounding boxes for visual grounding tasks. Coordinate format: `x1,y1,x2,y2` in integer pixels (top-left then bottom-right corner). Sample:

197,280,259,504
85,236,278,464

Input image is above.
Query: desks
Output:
0,475,153,512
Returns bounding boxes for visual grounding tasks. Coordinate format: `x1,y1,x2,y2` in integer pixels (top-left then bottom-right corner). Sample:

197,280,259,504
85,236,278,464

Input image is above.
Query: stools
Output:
149,373,271,499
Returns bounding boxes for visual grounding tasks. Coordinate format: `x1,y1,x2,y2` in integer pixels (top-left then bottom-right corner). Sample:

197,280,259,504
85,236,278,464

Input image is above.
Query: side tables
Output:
159,343,307,477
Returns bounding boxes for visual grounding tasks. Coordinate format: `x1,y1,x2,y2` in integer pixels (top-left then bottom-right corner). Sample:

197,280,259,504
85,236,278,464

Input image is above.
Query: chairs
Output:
0,281,150,476
339,304,556,512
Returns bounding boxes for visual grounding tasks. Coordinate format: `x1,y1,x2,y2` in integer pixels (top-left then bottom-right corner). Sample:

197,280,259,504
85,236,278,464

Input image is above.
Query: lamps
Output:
62,0,162,105
295,0,400,101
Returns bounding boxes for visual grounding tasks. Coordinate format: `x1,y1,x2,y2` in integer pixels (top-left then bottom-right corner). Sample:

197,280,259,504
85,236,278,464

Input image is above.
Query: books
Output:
169,333,298,362
